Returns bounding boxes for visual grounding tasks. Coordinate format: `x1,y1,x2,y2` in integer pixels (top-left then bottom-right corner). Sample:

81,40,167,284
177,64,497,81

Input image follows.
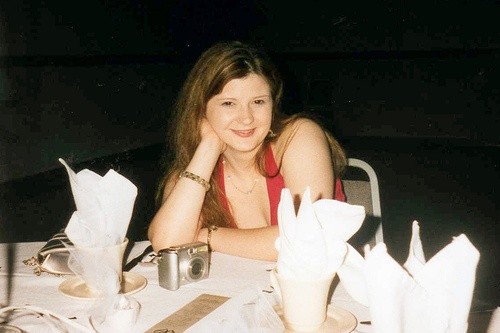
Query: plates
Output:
270,304,357,333
58,272,147,300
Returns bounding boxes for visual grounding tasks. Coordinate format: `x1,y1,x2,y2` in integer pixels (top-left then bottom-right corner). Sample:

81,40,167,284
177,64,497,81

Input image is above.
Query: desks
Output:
1,242,476,333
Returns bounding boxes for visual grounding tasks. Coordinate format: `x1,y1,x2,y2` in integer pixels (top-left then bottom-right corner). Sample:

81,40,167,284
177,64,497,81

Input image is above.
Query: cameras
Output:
157,241,210,290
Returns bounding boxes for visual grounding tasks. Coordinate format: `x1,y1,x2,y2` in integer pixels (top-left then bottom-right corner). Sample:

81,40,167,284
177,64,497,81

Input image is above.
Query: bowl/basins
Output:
104,300,140,328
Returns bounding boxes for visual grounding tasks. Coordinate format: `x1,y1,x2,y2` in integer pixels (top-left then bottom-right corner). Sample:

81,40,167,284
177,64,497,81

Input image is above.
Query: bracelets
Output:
179,171,210,192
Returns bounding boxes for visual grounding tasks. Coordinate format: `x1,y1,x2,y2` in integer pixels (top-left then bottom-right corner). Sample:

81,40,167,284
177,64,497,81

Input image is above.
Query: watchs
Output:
207,225,219,252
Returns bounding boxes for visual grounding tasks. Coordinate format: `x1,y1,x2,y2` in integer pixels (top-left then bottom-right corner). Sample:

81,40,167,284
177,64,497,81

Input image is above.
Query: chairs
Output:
335,158,384,252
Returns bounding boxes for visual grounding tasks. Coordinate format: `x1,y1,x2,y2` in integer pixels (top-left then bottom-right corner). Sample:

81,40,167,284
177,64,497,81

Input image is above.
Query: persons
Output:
147,40,348,261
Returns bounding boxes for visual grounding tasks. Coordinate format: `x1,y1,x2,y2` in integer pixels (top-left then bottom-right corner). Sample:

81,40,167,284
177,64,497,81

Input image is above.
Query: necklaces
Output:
222,161,259,194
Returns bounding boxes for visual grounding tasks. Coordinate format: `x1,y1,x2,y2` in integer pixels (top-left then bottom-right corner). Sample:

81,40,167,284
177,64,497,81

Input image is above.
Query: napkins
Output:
58,157,139,248
337,220,481,332
270,187,366,273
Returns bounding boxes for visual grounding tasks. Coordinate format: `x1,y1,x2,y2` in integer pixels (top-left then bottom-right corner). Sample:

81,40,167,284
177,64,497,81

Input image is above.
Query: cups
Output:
272,264,336,327
73,238,129,294
